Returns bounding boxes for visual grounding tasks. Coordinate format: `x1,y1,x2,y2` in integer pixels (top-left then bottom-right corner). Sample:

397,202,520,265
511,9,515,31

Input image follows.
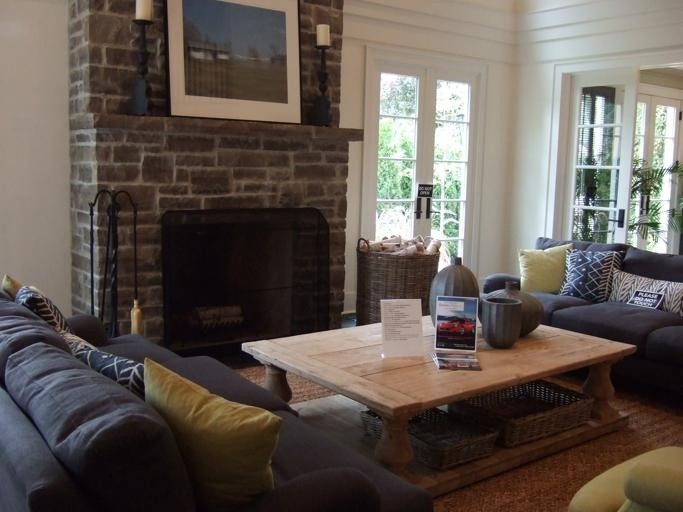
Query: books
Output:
433,352,482,371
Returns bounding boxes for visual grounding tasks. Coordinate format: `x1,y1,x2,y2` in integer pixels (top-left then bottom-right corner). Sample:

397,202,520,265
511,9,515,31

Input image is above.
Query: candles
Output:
131,1,154,21
315,23,331,46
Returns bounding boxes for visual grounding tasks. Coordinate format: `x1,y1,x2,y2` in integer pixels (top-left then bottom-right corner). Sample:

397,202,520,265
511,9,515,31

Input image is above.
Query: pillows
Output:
0,272,26,303
140,357,286,512
557,248,624,305
513,240,575,294
604,264,682,319
48,319,102,353
71,349,144,401
9,284,76,334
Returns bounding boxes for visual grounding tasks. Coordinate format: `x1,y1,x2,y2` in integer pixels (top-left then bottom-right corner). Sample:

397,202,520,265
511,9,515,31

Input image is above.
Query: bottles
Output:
429,257,479,326
358,235,441,255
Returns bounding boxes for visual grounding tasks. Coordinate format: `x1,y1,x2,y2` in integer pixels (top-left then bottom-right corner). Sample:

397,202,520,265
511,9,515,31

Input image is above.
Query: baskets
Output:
446,378,597,449
356,236,440,328
359,405,502,472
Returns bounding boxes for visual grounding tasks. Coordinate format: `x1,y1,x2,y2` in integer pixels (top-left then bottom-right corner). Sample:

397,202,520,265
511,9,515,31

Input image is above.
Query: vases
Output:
426,252,480,329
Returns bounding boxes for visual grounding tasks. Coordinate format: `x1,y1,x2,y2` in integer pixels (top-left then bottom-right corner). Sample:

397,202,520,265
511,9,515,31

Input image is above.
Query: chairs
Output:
564,442,682,512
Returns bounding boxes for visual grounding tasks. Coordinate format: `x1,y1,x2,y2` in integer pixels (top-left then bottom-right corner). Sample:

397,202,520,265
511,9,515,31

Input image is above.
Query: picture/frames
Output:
161,0,307,127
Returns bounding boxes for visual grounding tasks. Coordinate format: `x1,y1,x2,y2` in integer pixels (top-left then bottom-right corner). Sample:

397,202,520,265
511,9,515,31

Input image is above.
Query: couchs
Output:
0,290,433,511
478,234,681,396
